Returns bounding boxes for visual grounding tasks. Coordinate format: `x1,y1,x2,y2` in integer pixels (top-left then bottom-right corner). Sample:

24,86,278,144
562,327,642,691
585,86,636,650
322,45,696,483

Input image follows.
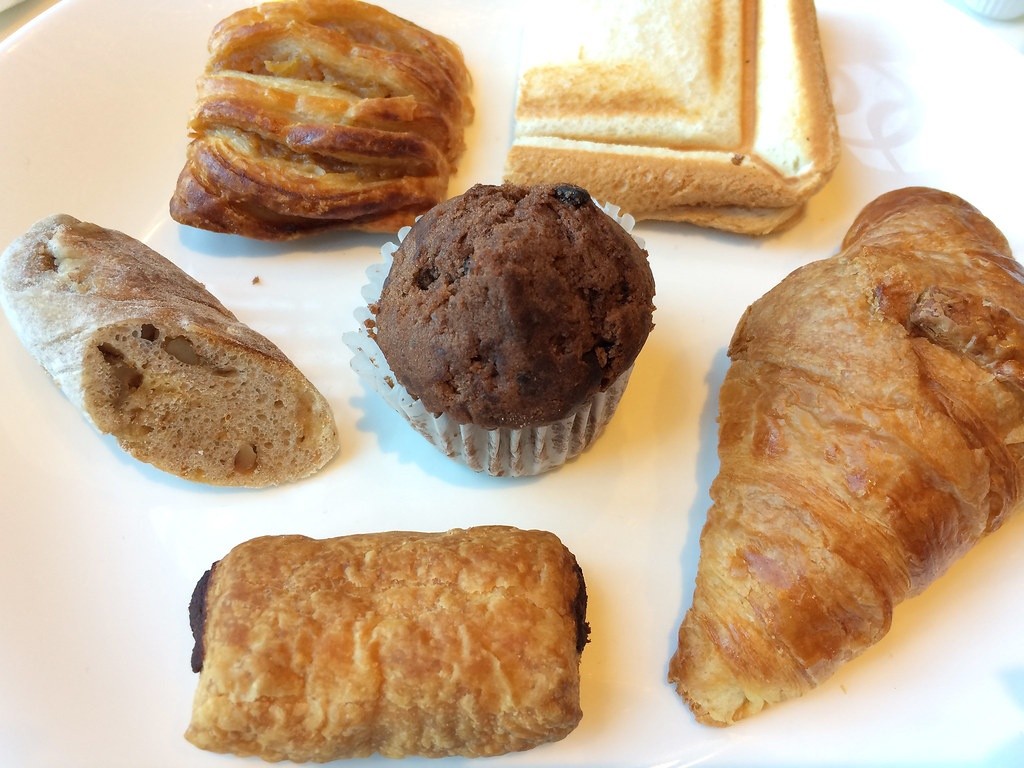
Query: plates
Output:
0,0,1024,768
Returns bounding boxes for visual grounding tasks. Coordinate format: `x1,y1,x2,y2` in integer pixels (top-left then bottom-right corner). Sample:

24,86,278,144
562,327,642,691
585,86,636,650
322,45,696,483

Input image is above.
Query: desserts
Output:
349,182,656,478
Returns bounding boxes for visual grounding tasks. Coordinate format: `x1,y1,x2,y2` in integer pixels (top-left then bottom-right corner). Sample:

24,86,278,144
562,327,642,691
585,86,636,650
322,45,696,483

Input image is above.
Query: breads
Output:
0,213,341,489
167,0,476,242
181,525,591,764
501,0,842,238
666,185,1024,728
